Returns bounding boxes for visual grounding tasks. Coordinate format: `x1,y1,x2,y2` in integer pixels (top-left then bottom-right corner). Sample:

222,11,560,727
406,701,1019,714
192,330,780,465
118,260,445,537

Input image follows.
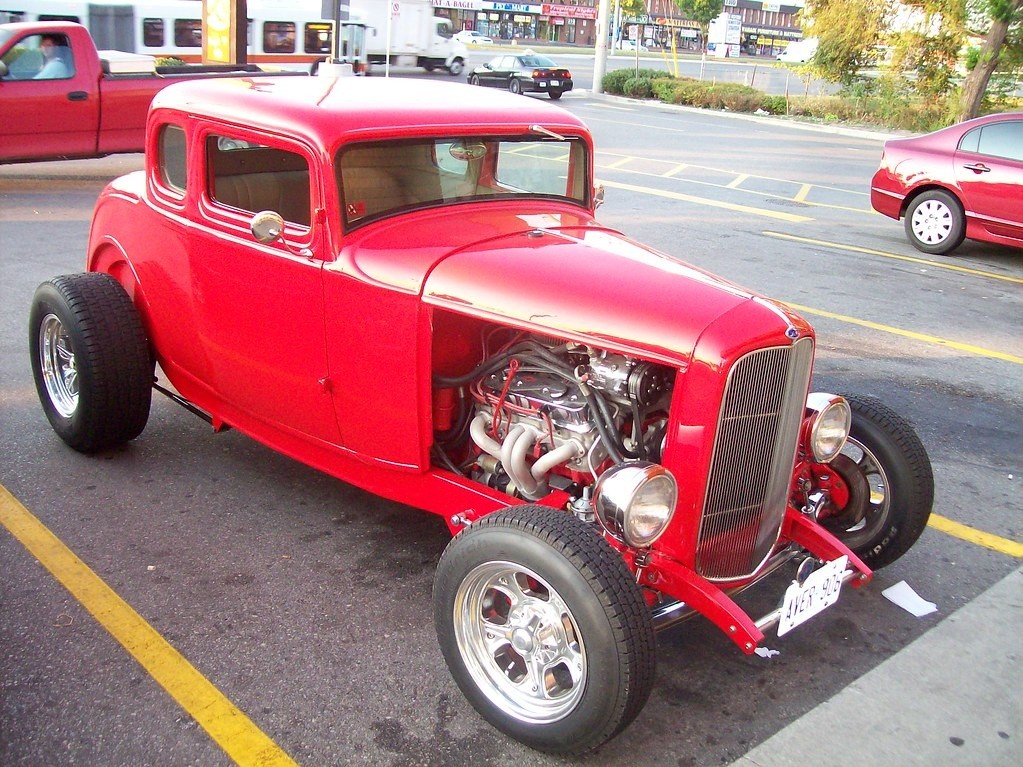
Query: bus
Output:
0,0,379,77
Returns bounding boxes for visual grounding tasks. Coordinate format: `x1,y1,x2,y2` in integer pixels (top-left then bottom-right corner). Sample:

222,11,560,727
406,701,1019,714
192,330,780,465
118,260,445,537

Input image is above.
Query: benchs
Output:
215,166,412,227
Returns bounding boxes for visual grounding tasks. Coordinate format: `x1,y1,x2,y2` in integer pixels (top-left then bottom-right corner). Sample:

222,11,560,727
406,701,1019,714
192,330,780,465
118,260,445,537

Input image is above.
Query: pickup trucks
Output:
0,20,272,166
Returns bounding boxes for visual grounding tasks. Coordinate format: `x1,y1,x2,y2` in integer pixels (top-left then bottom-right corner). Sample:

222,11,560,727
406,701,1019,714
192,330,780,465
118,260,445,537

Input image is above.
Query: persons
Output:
33,35,69,79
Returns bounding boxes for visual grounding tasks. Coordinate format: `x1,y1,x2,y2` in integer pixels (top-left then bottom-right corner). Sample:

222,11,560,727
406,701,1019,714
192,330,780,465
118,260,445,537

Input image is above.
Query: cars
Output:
616,39,648,52
30,76,936,759
466,52,573,100
453,31,493,45
870,110,1023,258
777,49,811,62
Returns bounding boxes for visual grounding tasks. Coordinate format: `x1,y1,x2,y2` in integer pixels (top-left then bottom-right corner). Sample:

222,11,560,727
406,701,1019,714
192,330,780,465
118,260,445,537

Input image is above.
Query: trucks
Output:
349,0,470,76
